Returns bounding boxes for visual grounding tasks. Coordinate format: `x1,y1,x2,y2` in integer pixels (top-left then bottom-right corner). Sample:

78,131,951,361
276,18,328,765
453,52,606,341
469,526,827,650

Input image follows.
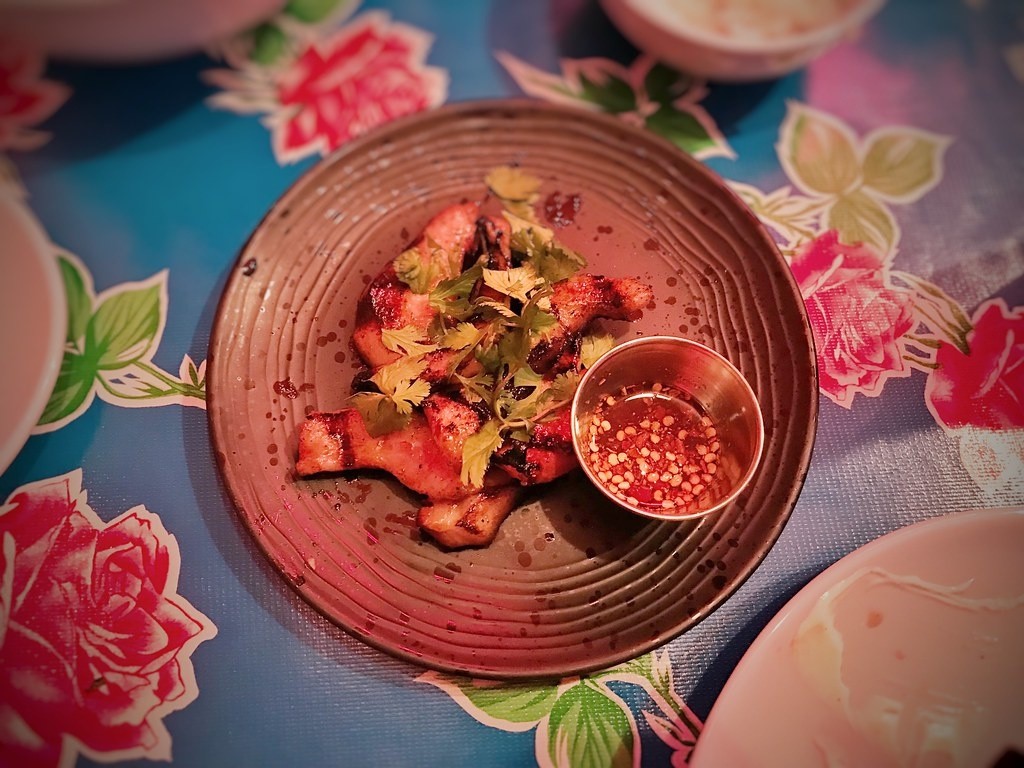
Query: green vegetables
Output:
343,163,616,489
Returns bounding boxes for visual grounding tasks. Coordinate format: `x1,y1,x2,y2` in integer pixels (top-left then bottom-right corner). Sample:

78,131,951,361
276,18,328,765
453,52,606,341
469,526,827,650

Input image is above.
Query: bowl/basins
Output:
604,0,885,79
571,336,765,522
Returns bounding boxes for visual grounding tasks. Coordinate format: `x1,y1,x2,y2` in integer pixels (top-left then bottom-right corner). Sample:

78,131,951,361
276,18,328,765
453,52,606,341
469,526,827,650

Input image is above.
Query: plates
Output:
688,508,1024,768
205,101,821,678
0,189,68,474
66,0,289,57
